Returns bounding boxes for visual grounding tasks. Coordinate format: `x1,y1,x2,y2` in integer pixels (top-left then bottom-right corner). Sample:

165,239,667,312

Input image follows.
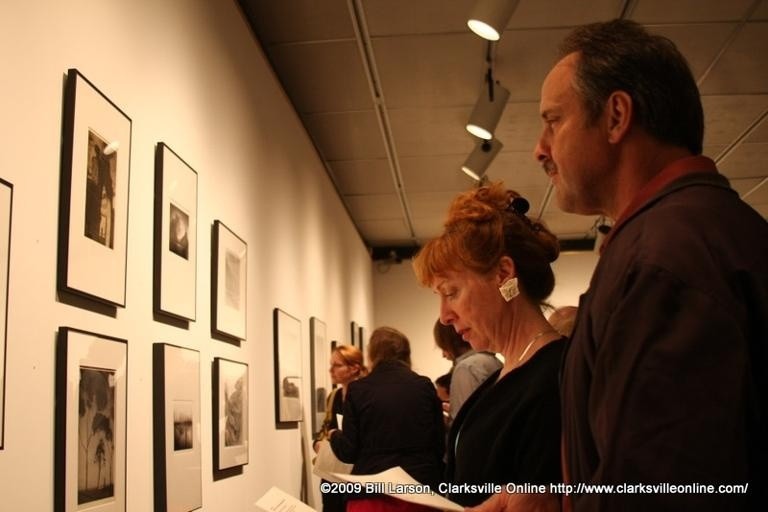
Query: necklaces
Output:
510,328,560,365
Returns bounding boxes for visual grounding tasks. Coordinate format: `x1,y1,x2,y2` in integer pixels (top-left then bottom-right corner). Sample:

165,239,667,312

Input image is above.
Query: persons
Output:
431,317,504,430
469,16,768,511
548,305,580,339
326,325,446,512
312,343,369,512
412,179,570,506
434,373,452,401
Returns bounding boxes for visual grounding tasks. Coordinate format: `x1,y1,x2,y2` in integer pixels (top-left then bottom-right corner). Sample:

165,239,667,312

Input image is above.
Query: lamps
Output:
465,38,511,141
461,138,503,182
468,0,520,41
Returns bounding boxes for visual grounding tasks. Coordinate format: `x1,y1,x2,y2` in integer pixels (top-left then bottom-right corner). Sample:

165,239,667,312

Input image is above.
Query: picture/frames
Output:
351,321,361,357
1,177,16,449
152,343,204,512
211,219,247,341
273,308,303,423
213,358,248,472
310,317,337,436
153,141,199,324
360,327,370,370
51,327,129,511
58,68,132,309
